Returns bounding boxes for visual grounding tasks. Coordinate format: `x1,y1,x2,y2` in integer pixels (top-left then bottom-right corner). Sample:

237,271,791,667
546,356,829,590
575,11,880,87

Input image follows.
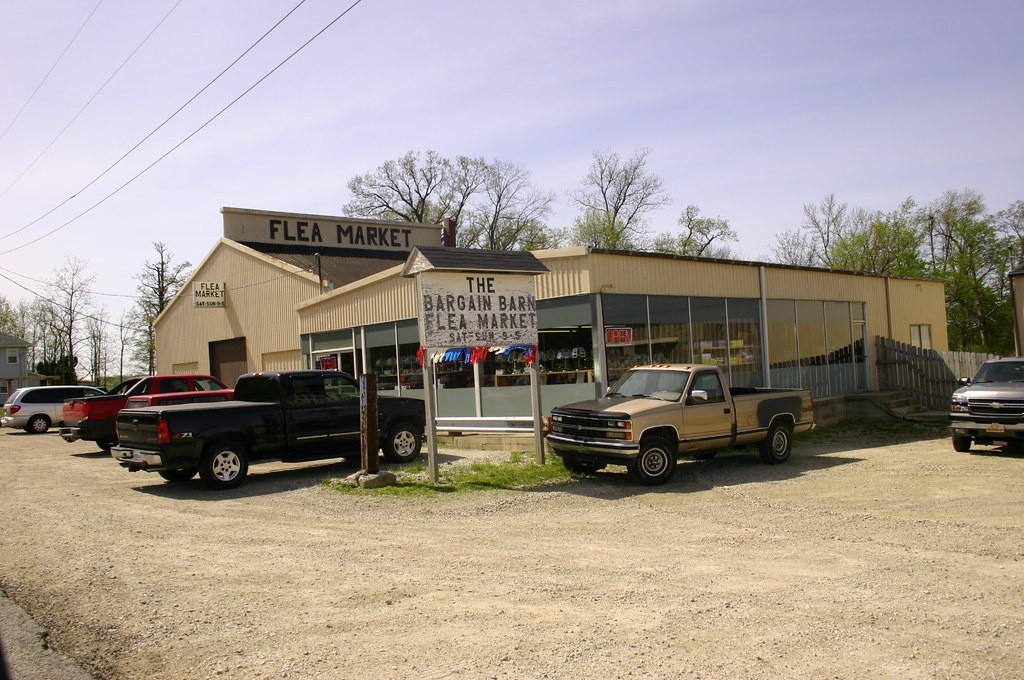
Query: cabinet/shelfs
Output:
695,343,756,371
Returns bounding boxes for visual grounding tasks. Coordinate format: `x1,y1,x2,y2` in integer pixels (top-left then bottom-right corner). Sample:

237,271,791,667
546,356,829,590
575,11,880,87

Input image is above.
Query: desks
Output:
495,370,594,386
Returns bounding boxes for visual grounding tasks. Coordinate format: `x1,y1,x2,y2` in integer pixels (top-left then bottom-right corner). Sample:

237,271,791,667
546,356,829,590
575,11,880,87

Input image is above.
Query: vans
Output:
4,386,107,434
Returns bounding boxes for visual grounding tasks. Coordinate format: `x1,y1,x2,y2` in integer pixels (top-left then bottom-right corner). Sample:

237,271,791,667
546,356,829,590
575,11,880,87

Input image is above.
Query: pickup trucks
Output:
110,369,424,490
948,357,1024,452
59,370,231,451
546,364,817,485
